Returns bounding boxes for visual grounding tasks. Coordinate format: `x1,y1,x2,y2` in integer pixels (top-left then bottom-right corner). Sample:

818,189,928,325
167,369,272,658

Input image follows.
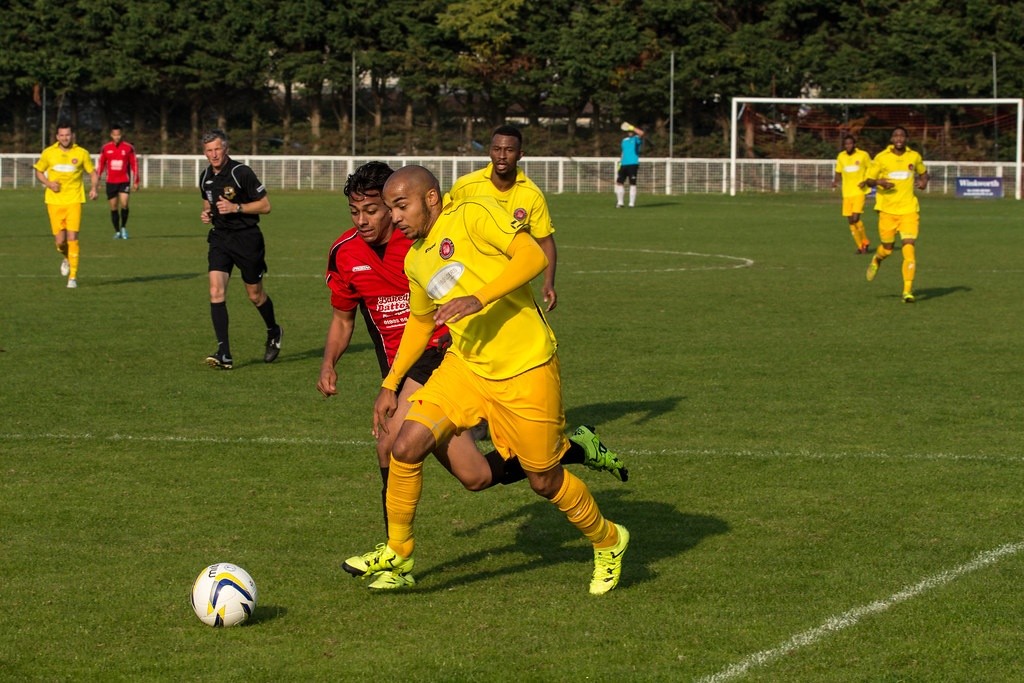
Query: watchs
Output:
237,203,242,213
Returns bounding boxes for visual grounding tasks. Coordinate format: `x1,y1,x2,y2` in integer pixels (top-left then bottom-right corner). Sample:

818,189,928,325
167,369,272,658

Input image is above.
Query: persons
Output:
317,161,629,575
199,130,282,371
452,126,558,313
614,123,644,208
342,164,629,594
865,127,927,302
832,135,871,253
33,122,99,288
96,127,140,240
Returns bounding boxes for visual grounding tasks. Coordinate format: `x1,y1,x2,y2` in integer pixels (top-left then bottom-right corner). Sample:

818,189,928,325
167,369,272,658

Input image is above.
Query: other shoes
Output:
615,204,625,209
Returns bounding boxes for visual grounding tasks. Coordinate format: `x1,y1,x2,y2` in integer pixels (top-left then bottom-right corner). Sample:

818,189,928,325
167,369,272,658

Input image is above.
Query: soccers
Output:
188,562,258,629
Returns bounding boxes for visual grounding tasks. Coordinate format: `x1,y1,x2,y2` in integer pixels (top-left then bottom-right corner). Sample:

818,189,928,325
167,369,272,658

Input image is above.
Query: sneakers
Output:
901,291,915,303
588,523,630,596
67,278,77,288
264,325,284,363
114,231,121,239
367,569,417,590
343,542,415,580
866,255,882,281
856,238,870,254
121,227,128,240
569,425,630,483
60,257,70,276
204,351,232,369
